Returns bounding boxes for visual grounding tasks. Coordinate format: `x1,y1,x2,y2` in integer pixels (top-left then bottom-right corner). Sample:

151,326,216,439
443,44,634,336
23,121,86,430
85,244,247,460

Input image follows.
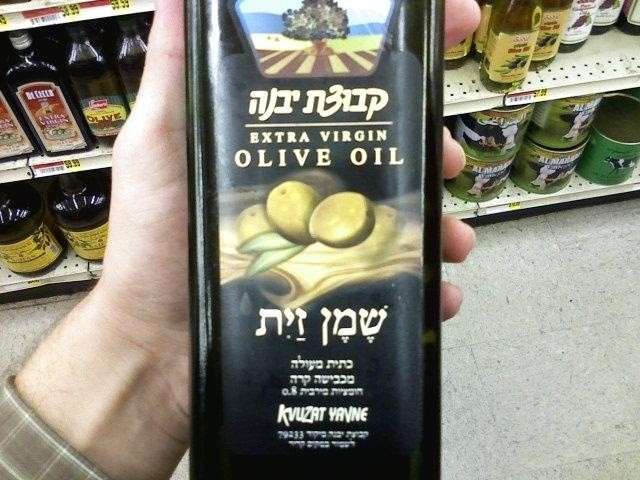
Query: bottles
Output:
0,14,152,278
183,0,445,478
444,0,640,94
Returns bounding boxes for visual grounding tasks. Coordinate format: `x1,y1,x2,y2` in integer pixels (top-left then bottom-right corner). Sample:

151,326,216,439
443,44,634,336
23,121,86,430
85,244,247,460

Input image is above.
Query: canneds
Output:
443,91,640,204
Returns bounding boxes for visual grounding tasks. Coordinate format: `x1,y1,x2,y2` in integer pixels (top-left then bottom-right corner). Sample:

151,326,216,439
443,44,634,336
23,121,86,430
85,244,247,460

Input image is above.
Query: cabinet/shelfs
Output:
0,1,640,293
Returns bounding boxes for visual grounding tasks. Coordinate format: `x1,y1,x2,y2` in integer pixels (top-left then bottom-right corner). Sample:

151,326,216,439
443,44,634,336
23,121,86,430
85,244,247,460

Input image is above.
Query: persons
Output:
0,0,479,478
